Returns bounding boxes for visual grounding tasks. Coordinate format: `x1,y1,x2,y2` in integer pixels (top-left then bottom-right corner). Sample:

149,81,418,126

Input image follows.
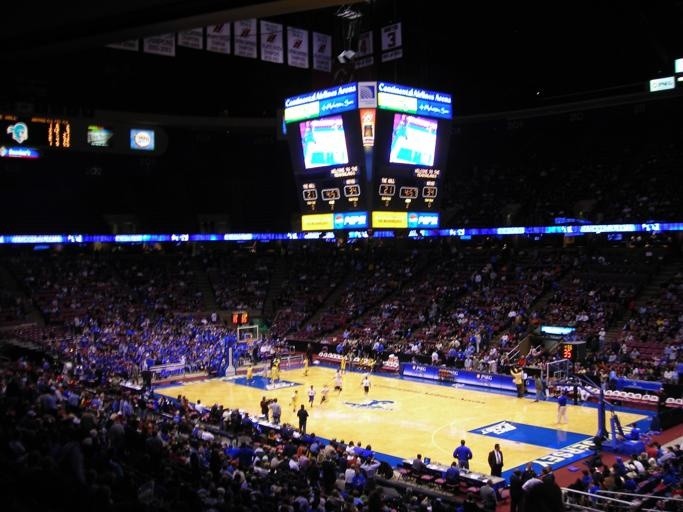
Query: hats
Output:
617,457,621,462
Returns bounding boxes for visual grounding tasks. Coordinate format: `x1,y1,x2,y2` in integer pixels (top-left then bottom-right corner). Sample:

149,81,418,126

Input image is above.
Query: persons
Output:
2,95,683,512
